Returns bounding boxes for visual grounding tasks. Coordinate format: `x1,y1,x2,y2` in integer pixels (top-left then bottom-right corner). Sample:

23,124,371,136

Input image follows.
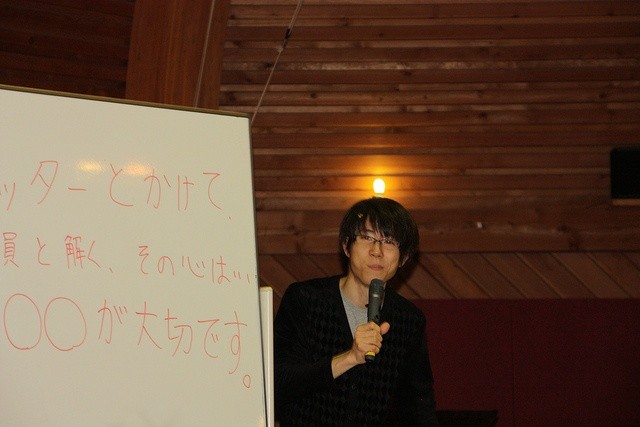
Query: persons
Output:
276,196,436,427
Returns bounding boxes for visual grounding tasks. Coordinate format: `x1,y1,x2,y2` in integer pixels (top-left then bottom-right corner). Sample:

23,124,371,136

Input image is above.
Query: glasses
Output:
354,234,401,248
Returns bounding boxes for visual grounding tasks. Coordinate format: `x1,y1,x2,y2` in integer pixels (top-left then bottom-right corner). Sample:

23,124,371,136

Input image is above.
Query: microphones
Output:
364,279,385,362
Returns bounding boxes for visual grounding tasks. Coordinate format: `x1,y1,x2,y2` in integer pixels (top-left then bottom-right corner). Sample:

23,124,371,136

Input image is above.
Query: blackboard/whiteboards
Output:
0,82,274,426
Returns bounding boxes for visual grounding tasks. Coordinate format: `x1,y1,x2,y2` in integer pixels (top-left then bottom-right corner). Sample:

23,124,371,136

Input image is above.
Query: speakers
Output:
610,147,640,206
